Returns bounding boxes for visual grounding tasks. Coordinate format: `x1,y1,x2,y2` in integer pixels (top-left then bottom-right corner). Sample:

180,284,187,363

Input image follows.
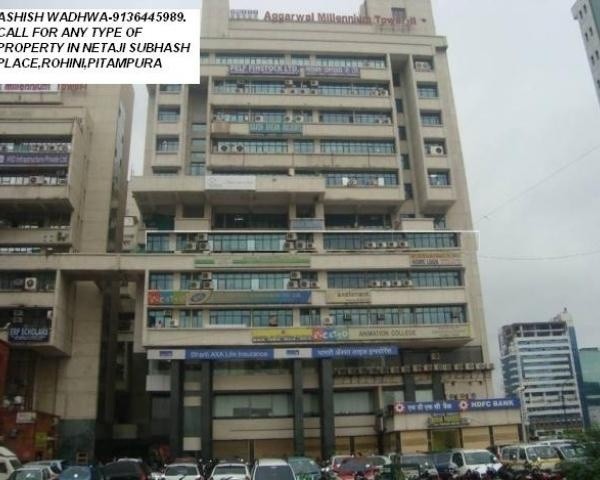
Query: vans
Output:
496,438,593,480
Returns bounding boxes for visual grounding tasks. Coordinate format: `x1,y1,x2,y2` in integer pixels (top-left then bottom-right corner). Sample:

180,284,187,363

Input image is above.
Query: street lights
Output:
561,373,579,431
